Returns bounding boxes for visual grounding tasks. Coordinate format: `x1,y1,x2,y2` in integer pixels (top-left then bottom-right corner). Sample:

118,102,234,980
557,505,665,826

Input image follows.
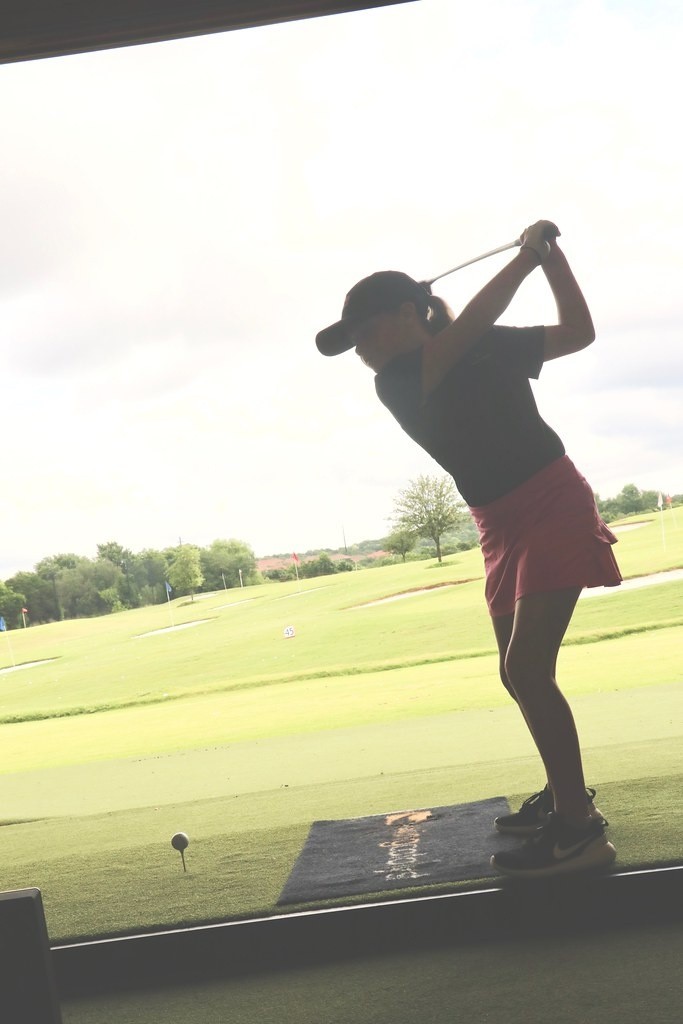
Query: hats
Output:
316,271,430,357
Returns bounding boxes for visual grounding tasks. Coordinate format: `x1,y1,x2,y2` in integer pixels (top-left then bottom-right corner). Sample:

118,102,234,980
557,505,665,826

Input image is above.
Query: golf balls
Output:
170,832,190,851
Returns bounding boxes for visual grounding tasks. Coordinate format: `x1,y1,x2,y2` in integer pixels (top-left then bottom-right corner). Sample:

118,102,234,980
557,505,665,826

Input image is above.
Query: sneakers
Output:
490,808,616,877
493,780,597,832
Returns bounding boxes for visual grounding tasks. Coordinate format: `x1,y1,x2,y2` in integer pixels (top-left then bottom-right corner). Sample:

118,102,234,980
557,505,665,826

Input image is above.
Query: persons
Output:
316,220,625,877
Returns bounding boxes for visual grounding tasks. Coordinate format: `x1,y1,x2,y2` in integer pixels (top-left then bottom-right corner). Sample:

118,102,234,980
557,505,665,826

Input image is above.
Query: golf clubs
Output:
314,224,558,357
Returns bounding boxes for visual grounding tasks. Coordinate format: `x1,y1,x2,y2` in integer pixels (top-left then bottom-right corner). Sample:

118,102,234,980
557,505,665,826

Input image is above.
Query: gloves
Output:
520,220,559,264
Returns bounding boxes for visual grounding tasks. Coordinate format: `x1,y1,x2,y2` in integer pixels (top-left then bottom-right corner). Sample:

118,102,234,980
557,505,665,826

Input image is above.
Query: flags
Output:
167,583,171,592
293,553,299,561
666,495,672,503
0,617,5,631
22,609,27,613
657,493,663,507
